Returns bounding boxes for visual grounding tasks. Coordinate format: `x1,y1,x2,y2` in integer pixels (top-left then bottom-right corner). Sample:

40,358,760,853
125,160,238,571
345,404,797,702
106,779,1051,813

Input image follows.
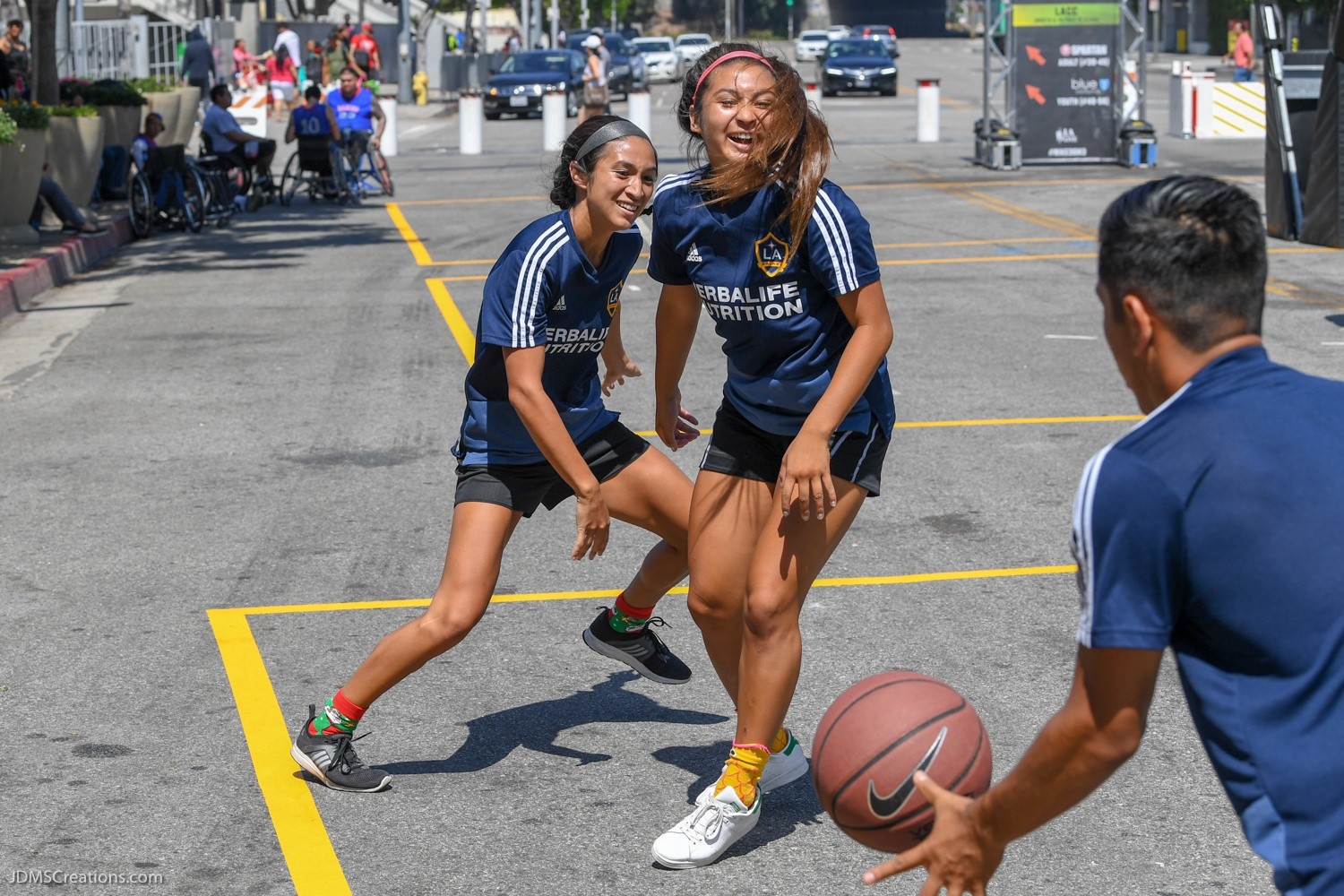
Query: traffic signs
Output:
1008,0,1119,164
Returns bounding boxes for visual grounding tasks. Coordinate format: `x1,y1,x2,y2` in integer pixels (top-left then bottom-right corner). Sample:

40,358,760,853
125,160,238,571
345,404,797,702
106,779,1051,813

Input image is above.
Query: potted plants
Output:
152,75,202,146
41,106,104,226
85,77,148,148
130,77,181,146
0,129,44,244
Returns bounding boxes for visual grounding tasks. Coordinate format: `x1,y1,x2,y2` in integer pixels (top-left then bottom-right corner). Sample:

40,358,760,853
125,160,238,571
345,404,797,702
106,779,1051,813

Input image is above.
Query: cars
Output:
483,48,588,120
849,25,897,56
815,38,900,97
630,36,685,84
675,33,720,66
827,24,852,42
792,29,831,62
565,27,642,101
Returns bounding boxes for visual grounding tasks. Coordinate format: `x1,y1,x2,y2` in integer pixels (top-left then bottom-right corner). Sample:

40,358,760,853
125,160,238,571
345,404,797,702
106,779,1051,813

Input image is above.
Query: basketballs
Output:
813,672,992,854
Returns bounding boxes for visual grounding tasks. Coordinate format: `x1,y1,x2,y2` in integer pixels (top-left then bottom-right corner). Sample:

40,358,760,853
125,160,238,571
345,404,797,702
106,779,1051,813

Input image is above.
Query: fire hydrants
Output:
412,71,428,106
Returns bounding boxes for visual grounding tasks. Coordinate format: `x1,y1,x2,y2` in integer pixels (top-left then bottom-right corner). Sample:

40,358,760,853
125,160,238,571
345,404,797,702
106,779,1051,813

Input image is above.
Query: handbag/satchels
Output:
584,56,610,107
354,35,369,68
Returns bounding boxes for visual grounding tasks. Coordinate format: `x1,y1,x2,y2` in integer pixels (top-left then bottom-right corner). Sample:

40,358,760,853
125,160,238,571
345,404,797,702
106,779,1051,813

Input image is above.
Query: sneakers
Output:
694,728,809,808
652,783,762,870
583,606,692,684
292,704,392,792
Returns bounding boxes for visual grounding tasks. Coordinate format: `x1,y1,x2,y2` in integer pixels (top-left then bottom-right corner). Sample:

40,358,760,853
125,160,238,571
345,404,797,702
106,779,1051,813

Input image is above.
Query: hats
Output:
362,23,374,34
581,34,601,49
591,27,604,37
147,114,165,132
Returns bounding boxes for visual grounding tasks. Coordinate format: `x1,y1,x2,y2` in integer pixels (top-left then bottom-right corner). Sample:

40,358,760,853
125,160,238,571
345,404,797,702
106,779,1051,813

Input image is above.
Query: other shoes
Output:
75,226,110,238
205,205,231,219
252,180,279,194
61,224,76,235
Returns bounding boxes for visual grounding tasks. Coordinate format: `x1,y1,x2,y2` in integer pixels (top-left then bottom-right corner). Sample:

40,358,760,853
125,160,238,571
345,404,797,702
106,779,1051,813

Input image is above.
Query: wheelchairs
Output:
128,143,206,240
195,130,276,216
279,142,394,207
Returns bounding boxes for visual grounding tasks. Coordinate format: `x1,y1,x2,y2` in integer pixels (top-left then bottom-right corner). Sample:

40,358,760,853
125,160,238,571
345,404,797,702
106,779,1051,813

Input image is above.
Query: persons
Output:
0,20,28,92
202,84,275,191
443,24,610,125
180,25,216,104
323,68,386,184
231,13,380,122
861,173,1344,896
1221,23,1256,82
644,44,895,867
130,113,185,222
290,115,692,794
29,161,109,236
284,86,342,199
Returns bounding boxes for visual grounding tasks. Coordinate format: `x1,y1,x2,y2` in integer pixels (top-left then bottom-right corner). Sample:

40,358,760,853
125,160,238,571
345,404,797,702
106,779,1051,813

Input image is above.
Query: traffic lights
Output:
786,0,794,8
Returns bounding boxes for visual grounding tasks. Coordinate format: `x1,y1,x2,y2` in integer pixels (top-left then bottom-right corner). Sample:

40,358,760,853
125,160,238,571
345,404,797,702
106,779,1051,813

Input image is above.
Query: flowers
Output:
0,99,50,130
